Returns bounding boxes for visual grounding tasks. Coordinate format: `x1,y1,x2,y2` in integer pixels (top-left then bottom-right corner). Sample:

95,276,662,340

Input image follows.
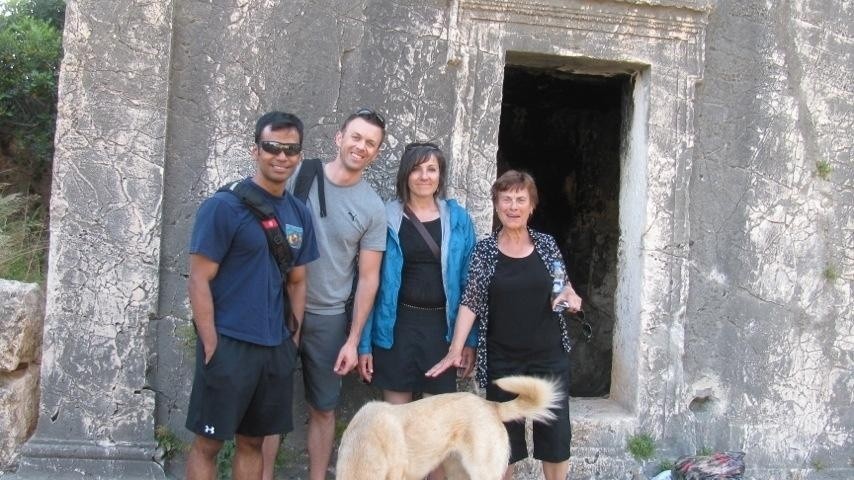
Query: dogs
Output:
334,374,565,480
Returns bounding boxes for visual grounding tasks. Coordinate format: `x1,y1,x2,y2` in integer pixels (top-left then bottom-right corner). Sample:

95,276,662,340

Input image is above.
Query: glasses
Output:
571,309,594,344
355,107,386,123
407,142,438,150
259,139,302,157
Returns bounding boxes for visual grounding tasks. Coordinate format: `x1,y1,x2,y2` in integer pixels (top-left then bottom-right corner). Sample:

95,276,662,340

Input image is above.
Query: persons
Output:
181,109,321,479
421,168,584,480
261,107,391,479
357,141,480,479
